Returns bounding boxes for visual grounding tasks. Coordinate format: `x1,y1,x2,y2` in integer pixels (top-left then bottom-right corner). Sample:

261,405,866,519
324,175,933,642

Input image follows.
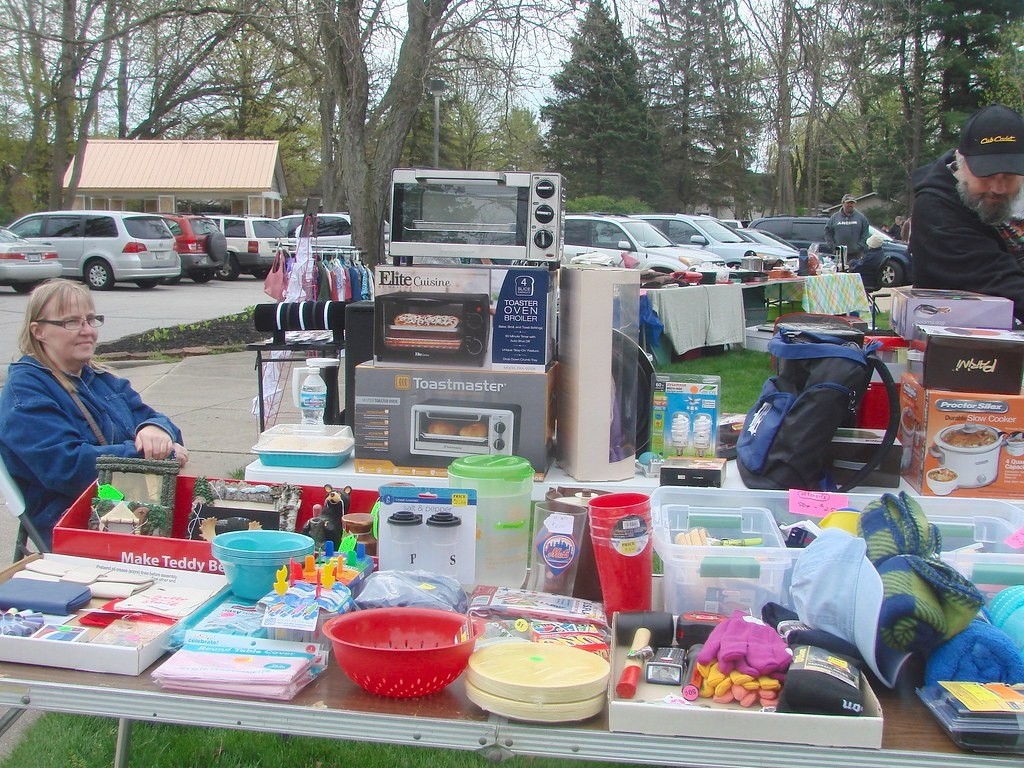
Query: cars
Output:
0,224,63,294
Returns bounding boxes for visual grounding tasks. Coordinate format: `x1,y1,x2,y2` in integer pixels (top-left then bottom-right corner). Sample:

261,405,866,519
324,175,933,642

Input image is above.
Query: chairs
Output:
864,255,890,315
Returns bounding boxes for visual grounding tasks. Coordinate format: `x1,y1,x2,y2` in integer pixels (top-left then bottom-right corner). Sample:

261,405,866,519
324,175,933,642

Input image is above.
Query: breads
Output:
427,421,488,436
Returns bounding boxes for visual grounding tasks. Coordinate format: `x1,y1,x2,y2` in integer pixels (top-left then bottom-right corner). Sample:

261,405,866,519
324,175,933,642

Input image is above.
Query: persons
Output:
911,102,1024,324
881,215,912,243
849,233,884,287
824,193,870,261
0,278,189,550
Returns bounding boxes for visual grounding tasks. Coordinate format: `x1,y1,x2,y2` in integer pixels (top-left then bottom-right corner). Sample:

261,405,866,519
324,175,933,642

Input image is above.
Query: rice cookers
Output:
900,406,916,468
928,424,1007,489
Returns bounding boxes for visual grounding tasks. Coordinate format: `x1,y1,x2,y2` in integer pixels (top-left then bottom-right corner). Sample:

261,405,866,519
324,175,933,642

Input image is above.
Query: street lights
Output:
430,75,446,167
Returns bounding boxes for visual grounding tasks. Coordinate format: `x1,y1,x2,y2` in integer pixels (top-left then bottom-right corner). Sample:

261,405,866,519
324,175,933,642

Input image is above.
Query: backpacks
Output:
734,327,901,492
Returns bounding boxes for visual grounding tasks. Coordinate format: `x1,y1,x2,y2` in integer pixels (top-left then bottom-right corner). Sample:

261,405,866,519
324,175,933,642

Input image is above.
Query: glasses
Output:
33,315,104,330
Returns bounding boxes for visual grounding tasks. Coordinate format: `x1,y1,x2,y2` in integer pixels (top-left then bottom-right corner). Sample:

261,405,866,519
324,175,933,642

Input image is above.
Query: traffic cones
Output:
716,216,825,276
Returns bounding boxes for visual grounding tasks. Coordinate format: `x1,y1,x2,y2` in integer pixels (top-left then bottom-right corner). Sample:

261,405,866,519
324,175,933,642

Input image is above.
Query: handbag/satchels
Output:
264,250,288,302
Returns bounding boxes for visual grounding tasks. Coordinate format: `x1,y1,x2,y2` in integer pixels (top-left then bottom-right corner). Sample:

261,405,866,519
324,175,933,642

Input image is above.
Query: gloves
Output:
696,609,791,708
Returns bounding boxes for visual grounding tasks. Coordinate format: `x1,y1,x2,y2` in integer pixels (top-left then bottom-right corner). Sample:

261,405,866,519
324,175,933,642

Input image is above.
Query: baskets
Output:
322,607,486,698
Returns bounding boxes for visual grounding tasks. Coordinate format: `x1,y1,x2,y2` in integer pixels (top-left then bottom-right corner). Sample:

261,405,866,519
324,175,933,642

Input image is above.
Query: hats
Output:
841,193,857,204
958,104,1024,178
866,235,883,248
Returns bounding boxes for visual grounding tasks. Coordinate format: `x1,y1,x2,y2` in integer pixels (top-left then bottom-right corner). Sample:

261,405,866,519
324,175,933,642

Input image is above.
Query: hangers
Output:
288,250,369,270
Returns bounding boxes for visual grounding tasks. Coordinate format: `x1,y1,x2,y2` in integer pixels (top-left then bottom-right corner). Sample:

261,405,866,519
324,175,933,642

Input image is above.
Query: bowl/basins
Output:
740,258,762,272
698,272,717,284
926,468,959,496
211,530,315,600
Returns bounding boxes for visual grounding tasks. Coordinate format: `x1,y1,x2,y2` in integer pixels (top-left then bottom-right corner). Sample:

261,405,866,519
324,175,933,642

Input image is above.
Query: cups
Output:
1006,433,1024,456
530,501,587,597
588,493,653,629
915,431,919,445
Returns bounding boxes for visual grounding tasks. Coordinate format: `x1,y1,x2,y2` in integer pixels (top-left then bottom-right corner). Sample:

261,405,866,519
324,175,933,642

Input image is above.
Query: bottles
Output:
426,513,462,578
341,513,377,556
299,367,327,424
798,249,808,276
387,510,423,571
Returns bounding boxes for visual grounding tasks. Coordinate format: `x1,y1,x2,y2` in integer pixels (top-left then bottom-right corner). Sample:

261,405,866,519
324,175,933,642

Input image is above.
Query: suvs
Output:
148,211,228,285
629,212,800,280
196,212,292,282
5,209,182,291
745,216,915,290
562,211,731,286
277,211,390,269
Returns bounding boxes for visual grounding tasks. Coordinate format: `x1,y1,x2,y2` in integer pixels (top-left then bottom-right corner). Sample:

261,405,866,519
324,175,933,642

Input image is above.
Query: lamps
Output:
617,252,640,269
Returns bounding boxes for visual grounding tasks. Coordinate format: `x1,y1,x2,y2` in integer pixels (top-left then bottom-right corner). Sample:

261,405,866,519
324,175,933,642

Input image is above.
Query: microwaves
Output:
410,398,521,460
374,290,490,367
388,168,567,271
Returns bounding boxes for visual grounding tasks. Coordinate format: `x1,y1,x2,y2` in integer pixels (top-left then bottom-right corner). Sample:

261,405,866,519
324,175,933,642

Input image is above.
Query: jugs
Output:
448,452,535,588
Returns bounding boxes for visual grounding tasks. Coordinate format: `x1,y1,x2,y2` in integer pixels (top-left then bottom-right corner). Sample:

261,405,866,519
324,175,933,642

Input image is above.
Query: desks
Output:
0,557,1024,768
613,273,860,350
246,331,346,433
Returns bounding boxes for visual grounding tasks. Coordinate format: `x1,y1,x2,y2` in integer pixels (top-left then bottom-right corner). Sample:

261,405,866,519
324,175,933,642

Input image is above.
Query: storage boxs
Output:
352,263,1024,623
51,472,378,577
